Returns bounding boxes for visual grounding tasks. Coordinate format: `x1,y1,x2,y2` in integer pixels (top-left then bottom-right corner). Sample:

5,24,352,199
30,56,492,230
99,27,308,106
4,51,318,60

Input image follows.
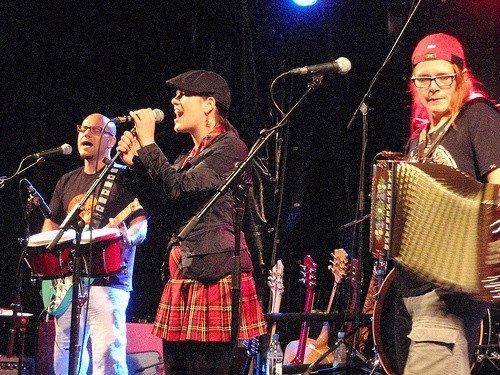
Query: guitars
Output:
289,256,316,367
346,258,364,365
265,260,285,364
284,249,348,366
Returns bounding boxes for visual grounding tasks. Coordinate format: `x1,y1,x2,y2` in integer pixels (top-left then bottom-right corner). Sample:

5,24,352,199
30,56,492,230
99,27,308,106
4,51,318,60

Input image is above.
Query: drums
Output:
24,229,76,279
79,228,124,276
371,268,500,375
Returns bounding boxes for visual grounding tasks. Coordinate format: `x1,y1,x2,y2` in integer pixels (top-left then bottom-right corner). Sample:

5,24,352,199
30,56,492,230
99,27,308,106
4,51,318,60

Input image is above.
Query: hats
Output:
411,32,468,71
166,70,232,108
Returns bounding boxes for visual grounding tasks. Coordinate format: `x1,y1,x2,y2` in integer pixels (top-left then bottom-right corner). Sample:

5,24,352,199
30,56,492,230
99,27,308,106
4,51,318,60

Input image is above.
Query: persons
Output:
41,113,152,375
115,69,266,374
393,33,500,375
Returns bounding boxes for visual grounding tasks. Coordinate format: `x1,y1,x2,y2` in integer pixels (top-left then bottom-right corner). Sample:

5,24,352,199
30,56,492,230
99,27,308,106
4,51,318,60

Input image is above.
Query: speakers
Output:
37,319,166,375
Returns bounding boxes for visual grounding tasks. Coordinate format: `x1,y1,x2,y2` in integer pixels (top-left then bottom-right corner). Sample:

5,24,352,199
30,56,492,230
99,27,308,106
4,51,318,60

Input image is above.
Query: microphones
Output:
22,179,52,219
32,143,72,159
112,108,165,122
290,56,351,75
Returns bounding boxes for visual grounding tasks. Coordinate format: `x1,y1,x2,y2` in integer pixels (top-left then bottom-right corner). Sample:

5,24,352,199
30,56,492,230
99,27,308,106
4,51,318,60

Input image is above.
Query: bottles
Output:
266,333,283,375
332,331,347,375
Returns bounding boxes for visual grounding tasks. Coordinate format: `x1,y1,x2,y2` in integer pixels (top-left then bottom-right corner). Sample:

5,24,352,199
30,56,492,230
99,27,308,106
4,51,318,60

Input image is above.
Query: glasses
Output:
76,124,114,138
412,68,468,91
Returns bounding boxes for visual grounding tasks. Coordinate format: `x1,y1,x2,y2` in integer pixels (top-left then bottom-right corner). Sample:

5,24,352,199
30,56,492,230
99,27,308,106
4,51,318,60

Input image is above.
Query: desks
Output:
37,320,165,375
